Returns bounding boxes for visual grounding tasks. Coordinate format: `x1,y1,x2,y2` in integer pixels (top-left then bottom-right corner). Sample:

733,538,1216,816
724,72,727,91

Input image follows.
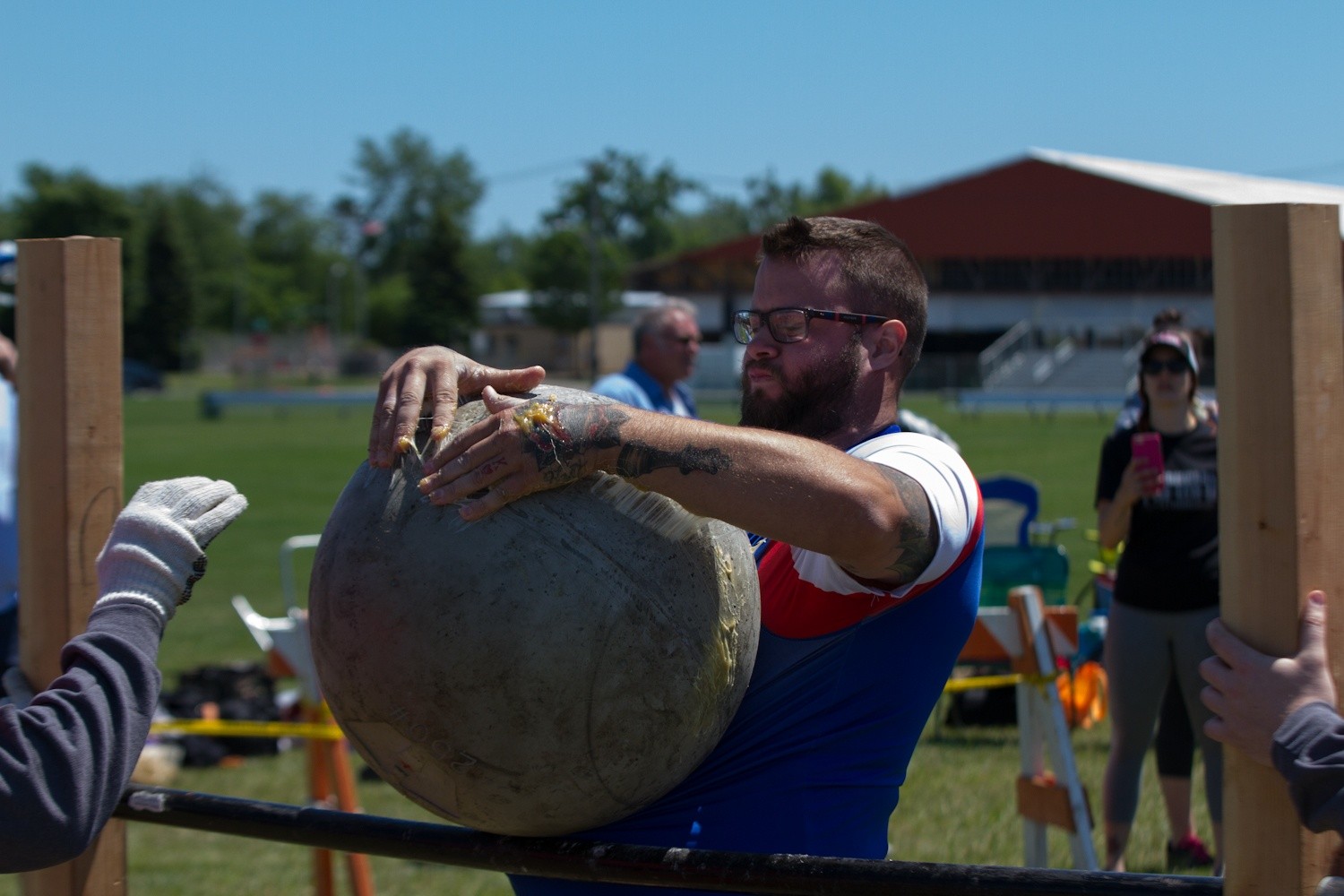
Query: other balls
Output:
309,380,761,835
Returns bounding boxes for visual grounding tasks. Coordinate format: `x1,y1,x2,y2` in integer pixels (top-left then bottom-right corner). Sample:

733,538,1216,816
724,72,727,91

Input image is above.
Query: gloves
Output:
93,476,250,621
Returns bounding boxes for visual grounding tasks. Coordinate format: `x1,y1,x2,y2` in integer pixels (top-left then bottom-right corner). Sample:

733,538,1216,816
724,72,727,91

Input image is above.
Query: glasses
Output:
661,334,704,345
731,306,913,355
1142,358,1190,375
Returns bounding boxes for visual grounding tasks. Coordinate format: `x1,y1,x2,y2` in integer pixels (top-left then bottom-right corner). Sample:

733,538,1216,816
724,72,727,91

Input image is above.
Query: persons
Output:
368,215,985,896
0,241,249,896
595,298,704,420
1092,313,1344,892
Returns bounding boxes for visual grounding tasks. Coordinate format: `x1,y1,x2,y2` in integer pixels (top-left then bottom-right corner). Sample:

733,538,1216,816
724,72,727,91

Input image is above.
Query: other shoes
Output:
1164,835,1215,872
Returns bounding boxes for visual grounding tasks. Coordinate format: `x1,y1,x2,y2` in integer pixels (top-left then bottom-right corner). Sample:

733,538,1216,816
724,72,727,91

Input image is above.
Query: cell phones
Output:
1132,432,1164,493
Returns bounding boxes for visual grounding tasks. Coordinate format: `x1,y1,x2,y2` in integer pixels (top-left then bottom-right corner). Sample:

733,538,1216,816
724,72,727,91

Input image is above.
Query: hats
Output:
1140,330,1200,376
